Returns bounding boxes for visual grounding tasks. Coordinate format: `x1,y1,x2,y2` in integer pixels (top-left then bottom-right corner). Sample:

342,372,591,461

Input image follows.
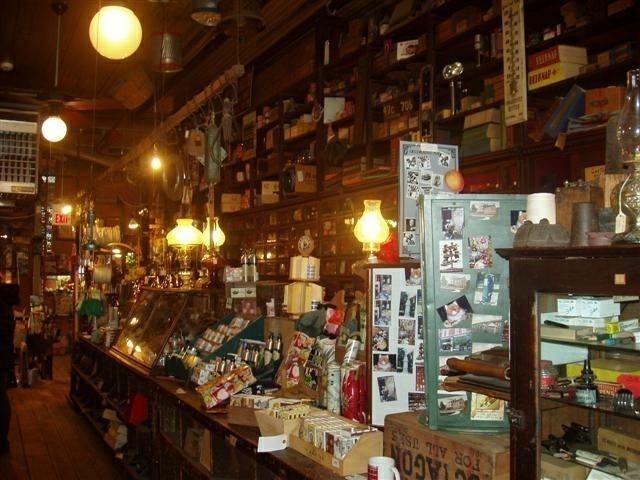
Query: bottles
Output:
217,356,226,375
271,336,281,365
259,333,274,368
171,335,177,353
227,357,236,372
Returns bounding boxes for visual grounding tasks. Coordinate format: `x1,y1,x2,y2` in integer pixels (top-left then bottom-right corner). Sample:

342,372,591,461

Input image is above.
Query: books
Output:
541,326,593,340
528,44,588,90
459,108,501,158
341,156,366,186
363,166,393,180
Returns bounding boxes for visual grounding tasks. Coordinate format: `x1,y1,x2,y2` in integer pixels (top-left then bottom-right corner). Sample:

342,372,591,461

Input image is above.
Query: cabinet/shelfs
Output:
192,0,640,276
70,275,640,480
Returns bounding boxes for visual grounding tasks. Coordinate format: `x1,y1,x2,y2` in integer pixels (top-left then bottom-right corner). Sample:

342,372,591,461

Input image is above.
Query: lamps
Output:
166,217,203,287
189,0,223,27
149,30,184,75
32,2,76,142
88,2,142,61
610,68,640,244
201,216,227,289
353,199,390,263
128,216,139,229
219,0,267,39
150,141,162,170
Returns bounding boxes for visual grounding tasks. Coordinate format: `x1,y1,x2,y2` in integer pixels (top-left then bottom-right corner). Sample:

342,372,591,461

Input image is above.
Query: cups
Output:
367,456,400,480
